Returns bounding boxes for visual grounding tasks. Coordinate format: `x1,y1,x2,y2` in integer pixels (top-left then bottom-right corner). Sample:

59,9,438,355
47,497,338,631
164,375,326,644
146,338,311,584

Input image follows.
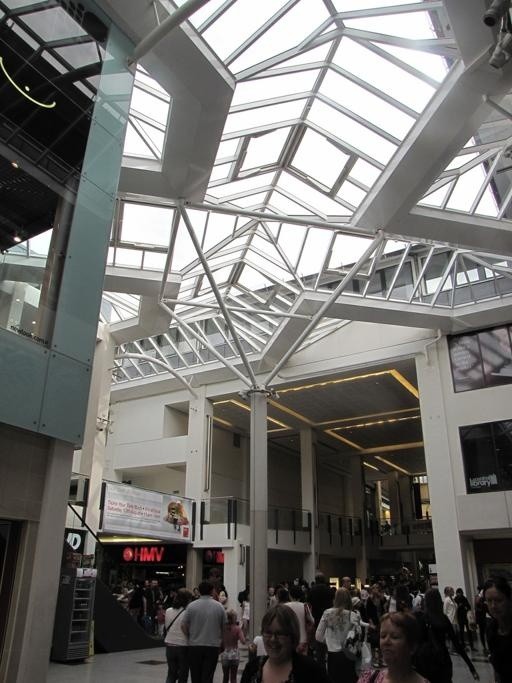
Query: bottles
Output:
75,579,89,630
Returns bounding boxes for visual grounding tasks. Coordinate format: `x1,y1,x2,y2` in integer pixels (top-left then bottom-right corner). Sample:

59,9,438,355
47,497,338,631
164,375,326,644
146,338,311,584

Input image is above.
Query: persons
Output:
381,520,392,536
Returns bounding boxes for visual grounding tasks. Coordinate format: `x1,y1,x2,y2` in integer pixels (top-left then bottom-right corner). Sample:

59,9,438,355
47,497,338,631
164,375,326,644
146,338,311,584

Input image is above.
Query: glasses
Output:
261,629,289,638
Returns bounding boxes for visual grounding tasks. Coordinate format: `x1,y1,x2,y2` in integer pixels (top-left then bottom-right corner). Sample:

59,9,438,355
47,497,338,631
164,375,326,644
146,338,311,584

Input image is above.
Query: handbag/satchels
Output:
342,626,361,661
304,602,315,634
413,613,453,683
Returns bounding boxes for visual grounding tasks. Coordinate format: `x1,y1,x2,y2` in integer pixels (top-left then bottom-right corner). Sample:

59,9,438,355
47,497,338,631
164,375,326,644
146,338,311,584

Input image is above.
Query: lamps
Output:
483,0,511,67
94,414,115,434
237,385,281,402
